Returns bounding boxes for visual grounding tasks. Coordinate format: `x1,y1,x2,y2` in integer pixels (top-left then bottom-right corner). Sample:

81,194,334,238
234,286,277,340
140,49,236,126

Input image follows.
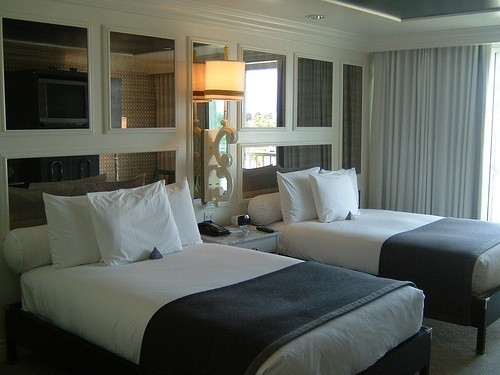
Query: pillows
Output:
3,224,53,273
43,191,102,270
87,178,183,267
166,178,204,246
248,165,358,223
6,175,145,220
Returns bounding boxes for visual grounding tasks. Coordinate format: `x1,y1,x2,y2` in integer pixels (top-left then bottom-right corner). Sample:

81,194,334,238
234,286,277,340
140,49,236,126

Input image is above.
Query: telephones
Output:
197,220,231,237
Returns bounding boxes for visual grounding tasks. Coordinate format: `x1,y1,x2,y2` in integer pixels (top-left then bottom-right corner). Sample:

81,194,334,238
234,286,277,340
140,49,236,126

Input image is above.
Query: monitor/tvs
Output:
38,78,89,129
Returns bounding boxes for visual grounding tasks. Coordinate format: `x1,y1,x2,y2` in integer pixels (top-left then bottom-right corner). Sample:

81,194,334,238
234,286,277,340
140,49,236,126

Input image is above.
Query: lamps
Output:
190,60,212,103
205,57,245,100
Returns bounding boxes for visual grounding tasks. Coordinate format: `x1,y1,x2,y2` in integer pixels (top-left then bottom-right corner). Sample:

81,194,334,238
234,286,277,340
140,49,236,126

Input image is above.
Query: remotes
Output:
256,225,274,233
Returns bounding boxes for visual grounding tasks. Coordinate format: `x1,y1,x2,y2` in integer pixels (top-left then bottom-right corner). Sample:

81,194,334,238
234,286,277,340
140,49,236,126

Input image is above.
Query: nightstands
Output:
201,223,280,251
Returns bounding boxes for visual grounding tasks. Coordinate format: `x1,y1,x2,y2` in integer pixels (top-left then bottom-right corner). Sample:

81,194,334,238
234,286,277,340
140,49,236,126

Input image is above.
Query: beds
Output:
24,239,432,375
258,209,500,354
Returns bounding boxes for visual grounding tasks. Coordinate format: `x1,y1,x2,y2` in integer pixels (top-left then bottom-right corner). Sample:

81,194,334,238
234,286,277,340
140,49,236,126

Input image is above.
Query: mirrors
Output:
2,17,360,229
